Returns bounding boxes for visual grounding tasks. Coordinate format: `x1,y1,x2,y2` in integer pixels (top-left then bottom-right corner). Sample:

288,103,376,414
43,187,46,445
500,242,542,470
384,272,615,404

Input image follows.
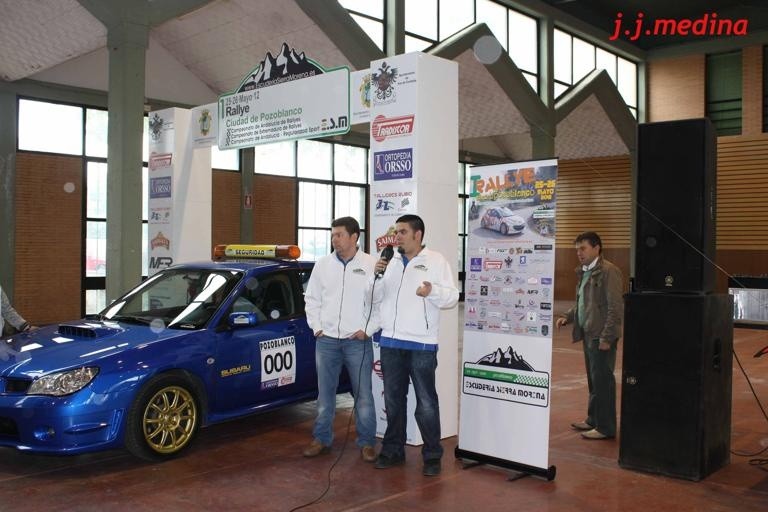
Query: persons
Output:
372,214,460,476
0,337,31,364
0,284,43,338
555,231,627,440
302,216,380,462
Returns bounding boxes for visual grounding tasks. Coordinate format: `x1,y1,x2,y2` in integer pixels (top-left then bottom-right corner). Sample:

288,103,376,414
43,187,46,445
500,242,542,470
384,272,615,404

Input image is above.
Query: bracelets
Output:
24,325,31,333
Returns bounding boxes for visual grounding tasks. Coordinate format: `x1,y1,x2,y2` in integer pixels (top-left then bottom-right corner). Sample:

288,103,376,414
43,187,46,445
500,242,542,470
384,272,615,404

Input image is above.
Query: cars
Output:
0,245,354,460
480,206,527,236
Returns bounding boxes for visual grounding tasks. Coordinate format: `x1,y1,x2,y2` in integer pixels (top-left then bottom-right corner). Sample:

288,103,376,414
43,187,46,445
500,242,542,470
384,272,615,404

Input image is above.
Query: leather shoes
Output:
581,428,609,438
304,440,331,457
375,457,398,469
424,459,441,476
571,421,592,429
360,446,377,463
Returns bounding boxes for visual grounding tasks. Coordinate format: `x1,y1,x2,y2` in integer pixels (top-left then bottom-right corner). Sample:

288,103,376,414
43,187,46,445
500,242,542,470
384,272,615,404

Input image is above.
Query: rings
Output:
377,264,379,267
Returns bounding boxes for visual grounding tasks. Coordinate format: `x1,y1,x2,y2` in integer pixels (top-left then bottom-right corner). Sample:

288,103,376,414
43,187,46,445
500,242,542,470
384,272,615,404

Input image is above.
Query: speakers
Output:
616,292,734,483
633,117,719,294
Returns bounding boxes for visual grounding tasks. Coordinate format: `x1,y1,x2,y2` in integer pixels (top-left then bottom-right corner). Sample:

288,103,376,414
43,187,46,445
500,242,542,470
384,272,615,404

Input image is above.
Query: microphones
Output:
375,242,394,280
